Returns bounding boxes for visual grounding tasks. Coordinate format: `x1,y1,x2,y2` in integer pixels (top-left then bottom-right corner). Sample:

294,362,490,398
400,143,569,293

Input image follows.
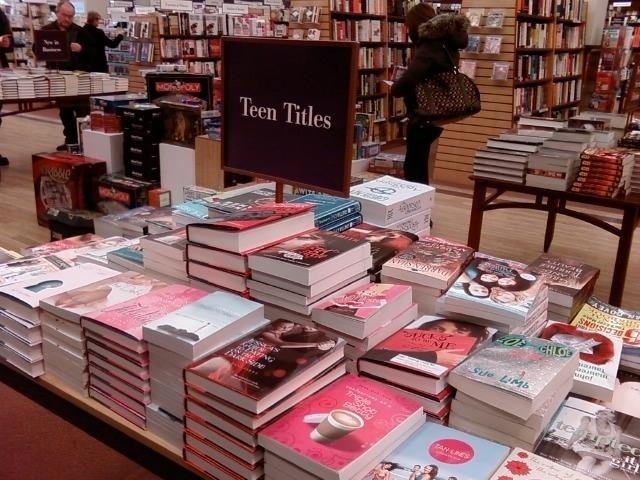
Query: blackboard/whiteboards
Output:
220,37,360,198
146,73,213,111
34,30,71,61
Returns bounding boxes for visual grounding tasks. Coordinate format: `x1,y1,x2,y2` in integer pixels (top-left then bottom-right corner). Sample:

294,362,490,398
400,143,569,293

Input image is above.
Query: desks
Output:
460,175,640,310
0,91,127,166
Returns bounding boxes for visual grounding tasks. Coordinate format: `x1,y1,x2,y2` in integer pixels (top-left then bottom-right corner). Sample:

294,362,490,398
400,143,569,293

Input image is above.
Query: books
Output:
0,0,640,480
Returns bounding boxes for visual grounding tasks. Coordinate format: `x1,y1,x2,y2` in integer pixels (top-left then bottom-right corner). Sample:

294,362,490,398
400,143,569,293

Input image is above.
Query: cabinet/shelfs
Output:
118,0,639,191
0,0,51,72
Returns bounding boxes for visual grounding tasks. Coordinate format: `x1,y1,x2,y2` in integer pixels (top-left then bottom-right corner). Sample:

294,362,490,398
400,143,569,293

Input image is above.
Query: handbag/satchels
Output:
411,70,482,120
